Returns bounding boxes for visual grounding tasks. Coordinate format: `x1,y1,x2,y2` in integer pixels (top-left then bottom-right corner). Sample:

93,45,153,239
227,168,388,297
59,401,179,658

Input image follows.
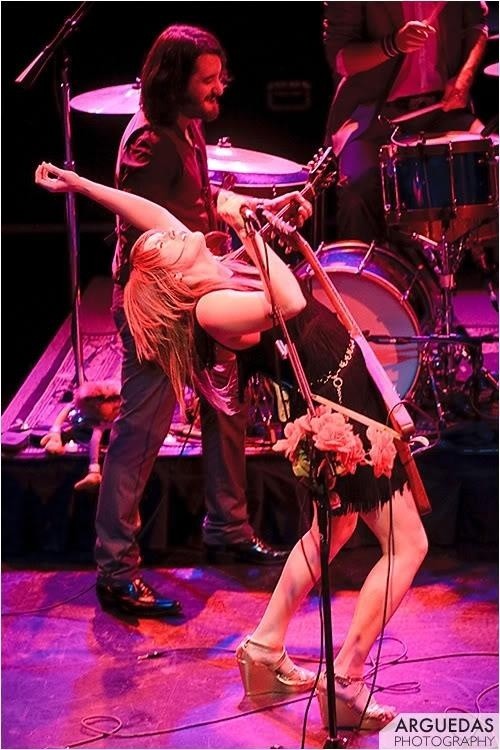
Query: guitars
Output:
263,211,432,516
204,120,358,264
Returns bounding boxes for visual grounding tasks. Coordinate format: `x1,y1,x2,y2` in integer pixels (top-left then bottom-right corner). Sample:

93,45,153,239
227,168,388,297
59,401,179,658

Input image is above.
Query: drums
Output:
204,143,311,265
378,131,499,226
291,240,437,404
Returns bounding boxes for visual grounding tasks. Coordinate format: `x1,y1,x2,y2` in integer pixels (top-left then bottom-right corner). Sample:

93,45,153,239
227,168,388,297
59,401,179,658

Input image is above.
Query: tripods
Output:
398,219,499,431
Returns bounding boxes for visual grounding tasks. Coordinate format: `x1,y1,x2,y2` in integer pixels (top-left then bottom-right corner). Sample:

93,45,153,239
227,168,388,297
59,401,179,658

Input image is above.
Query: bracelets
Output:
376,30,401,61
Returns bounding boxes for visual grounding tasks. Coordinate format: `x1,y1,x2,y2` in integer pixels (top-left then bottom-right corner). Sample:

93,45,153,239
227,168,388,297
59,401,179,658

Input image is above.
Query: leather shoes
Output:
198,538,288,564
96,578,183,616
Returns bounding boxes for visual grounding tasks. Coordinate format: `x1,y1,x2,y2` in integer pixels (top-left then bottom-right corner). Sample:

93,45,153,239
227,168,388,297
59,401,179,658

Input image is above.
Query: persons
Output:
34,161,433,732
95,22,295,622
316,2,499,251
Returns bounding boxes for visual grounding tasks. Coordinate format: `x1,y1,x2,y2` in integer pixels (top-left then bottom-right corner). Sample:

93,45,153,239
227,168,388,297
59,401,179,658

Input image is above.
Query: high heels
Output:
236,635,397,729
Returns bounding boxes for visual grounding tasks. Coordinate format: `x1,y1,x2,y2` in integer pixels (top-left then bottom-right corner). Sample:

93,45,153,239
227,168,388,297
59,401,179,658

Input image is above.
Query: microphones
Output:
214,188,256,223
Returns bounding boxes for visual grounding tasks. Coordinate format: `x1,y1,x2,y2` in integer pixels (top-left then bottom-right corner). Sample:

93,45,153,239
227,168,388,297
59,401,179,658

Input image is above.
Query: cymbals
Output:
484,63,499,76
69,83,142,114
